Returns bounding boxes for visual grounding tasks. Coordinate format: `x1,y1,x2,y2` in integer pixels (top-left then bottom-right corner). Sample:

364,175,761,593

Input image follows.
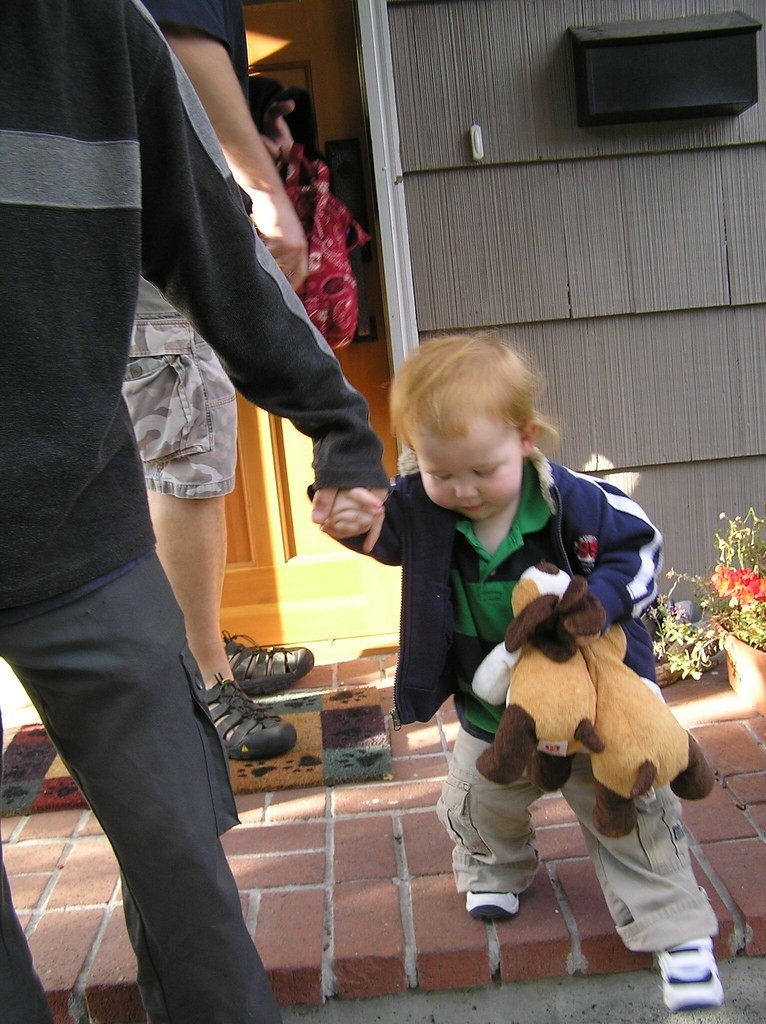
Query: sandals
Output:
203,673,297,762
221,629,314,695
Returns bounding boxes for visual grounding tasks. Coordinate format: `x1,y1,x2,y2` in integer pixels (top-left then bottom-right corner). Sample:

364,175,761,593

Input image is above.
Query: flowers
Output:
640,569,712,681
710,508,766,652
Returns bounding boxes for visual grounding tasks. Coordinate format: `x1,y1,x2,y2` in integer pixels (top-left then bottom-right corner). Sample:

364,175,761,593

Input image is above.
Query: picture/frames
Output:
247,59,321,156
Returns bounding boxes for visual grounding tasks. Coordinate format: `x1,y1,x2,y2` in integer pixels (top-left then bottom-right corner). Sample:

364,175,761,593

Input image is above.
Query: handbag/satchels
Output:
274,139,371,349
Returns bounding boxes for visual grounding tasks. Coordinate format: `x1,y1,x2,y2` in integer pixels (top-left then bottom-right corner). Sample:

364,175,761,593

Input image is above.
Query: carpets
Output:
0,682,392,818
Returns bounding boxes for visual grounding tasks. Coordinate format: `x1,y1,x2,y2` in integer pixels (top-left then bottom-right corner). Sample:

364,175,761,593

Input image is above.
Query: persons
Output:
320,339,723,1013
120,0,315,763
0,0,388,1024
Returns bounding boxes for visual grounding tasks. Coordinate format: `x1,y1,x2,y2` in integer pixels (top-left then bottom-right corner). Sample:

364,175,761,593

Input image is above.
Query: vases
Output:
711,615,766,718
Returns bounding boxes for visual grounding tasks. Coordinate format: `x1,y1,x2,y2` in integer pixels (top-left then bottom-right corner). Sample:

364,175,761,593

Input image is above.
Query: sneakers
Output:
656,934,724,1010
465,890,519,919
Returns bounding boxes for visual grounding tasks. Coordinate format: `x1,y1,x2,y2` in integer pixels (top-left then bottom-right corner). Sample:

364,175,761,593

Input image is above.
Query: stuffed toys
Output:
471,561,715,839
